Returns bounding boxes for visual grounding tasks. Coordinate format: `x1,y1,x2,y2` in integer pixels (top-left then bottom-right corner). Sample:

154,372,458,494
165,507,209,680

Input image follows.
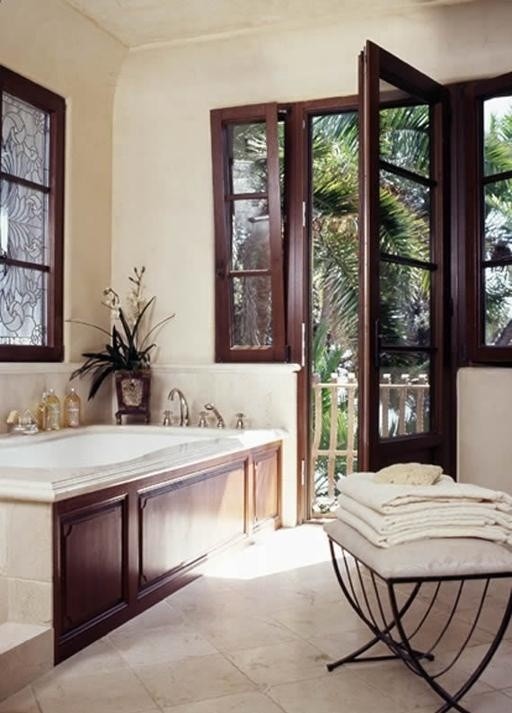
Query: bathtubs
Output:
0,425,285,667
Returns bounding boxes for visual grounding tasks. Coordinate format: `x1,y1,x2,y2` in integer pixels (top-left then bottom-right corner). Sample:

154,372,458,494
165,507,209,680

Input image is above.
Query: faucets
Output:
168,387,190,427
205,403,224,427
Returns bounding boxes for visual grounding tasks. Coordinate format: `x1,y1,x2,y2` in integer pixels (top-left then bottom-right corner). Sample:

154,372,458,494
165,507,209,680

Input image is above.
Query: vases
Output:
113,372,149,422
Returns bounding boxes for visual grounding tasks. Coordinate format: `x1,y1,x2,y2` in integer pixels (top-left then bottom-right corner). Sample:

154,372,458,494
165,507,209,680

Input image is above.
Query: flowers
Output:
65,264,179,400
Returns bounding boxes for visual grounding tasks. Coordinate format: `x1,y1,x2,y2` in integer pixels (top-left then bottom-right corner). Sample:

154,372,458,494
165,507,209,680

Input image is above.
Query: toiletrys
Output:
45,388,62,431
64,387,81,428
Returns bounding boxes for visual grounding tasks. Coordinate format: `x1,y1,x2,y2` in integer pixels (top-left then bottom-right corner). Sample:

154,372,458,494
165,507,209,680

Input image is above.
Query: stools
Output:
323,521,511,712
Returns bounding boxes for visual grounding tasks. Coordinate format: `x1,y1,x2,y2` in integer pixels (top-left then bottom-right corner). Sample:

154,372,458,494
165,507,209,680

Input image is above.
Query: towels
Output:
330,458,511,550
